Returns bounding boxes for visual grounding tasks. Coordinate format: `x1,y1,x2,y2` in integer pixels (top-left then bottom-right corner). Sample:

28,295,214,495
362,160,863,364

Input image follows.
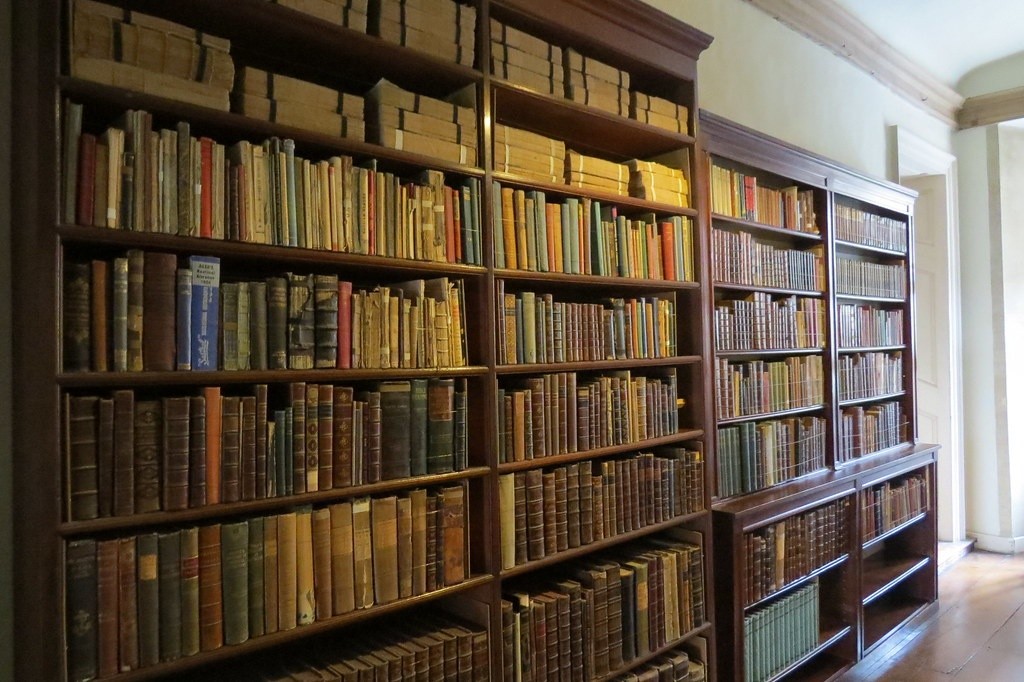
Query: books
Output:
67,0,931,682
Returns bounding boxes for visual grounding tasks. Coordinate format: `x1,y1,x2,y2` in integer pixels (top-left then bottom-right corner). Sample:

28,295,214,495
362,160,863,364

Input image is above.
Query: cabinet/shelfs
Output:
0,0,941,682
